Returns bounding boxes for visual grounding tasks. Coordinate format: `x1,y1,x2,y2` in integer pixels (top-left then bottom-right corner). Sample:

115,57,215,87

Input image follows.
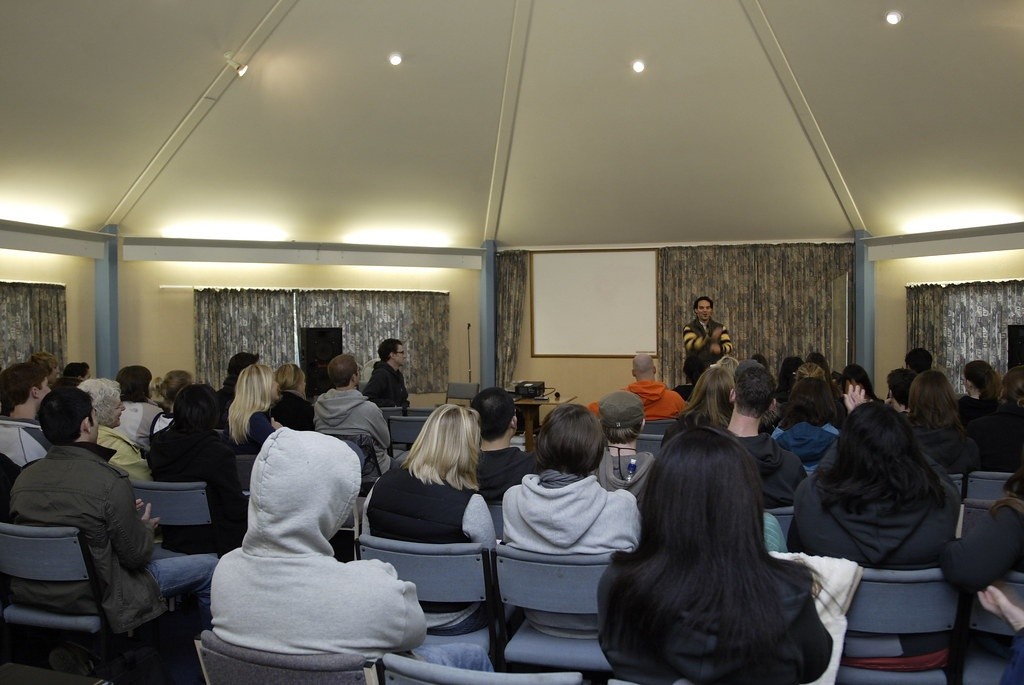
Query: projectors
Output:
514,381,546,398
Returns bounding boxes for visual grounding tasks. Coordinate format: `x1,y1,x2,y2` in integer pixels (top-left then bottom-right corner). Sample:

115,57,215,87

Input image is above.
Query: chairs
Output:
0,384,1024,685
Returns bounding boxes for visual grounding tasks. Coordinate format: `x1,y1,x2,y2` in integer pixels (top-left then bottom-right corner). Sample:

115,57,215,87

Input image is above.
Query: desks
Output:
515,395,577,454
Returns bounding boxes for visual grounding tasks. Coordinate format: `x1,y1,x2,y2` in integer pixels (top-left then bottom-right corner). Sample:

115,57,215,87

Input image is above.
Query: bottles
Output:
623,459,637,480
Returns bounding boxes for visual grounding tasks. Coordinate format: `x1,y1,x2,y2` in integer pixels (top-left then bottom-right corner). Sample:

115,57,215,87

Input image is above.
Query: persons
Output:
0,339,1024,685
681,296,734,385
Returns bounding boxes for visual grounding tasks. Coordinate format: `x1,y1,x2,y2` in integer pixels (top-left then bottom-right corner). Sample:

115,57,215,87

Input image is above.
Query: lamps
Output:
224,51,249,78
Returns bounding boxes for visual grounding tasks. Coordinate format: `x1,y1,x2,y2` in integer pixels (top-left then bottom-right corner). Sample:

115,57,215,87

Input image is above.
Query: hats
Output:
599,391,643,429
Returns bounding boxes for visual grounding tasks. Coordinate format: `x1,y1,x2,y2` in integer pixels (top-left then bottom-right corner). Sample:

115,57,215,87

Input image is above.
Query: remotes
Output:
534,397,549,400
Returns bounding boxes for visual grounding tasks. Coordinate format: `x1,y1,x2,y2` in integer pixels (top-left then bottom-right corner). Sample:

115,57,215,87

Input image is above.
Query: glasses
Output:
960,378,967,386
393,350,405,354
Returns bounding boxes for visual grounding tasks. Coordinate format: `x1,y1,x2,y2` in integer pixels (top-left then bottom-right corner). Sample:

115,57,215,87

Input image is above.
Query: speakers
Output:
301,327,343,397
1008,325,1024,372
